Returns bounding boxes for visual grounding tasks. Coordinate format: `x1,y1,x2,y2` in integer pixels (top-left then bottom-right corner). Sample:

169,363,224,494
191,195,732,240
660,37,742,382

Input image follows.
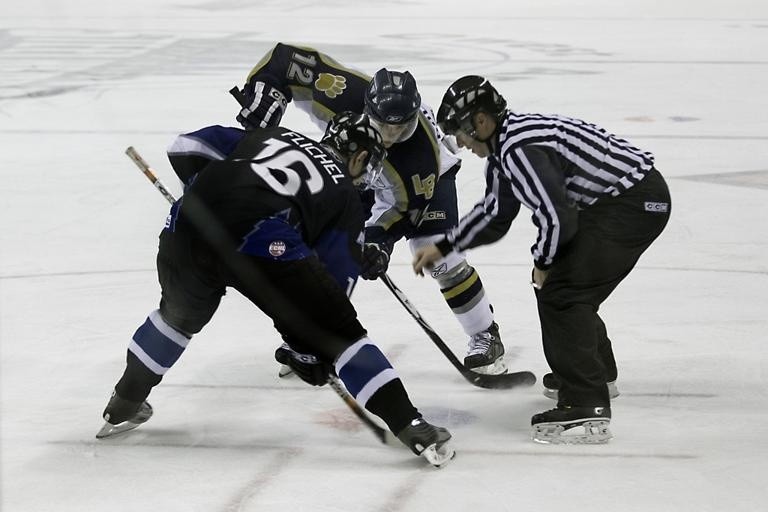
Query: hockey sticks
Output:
124,147,404,446
229,86,536,389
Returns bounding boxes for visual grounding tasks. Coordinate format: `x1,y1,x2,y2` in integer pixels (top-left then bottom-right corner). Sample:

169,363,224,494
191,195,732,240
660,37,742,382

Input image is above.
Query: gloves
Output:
236,72,292,129
275,343,329,386
361,226,394,280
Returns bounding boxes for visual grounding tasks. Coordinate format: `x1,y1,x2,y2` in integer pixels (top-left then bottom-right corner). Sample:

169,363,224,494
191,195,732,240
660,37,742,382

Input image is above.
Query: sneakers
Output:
464,304,503,368
103,390,153,424
397,418,451,456
532,341,617,425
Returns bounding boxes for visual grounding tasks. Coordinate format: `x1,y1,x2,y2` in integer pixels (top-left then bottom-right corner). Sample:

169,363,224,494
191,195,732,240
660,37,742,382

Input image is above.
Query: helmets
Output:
437,76,506,155
319,68,421,191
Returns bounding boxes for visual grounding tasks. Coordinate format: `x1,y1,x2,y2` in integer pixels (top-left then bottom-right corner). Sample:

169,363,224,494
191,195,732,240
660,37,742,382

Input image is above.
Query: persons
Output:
411,74,671,426
100,110,455,455
236,43,504,368
